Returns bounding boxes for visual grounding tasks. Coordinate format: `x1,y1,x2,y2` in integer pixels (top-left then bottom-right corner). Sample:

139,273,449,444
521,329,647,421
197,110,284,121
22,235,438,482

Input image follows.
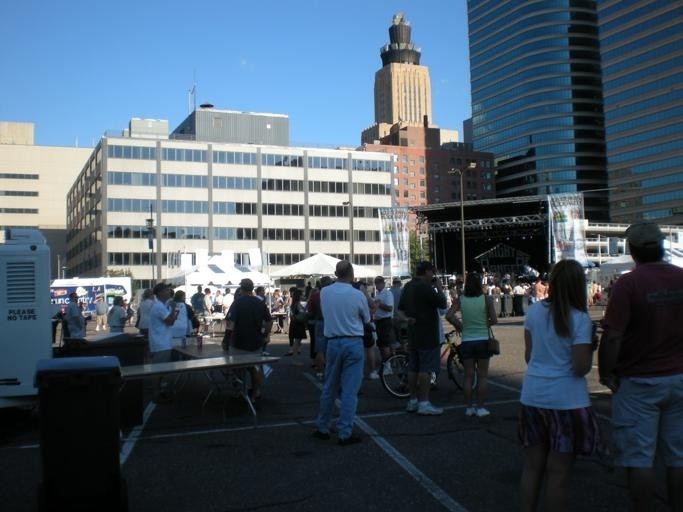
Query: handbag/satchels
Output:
488,326,500,355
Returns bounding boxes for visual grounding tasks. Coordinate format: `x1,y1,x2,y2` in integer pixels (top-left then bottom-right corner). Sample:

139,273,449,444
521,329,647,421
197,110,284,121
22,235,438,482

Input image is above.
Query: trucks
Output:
51,278,132,316
0,229,51,412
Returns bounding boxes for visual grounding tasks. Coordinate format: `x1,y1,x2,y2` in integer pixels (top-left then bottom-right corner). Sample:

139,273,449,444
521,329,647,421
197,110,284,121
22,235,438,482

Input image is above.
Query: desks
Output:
118,354,281,432
173,343,254,405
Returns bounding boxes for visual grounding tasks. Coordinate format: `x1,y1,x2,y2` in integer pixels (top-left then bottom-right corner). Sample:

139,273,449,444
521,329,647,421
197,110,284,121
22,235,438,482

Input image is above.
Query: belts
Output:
326,336,363,339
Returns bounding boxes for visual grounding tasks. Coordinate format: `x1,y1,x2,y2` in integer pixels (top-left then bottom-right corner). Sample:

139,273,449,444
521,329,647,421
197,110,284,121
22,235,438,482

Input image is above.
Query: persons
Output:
443,272,498,418
395,260,448,416
221,277,273,405
310,259,376,448
596,219,682,512
514,258,599,511
47,257,619,390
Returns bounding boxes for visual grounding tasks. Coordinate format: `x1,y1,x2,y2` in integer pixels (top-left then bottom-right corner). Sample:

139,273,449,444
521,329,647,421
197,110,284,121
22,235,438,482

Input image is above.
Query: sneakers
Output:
407,398,443,415
370,368,393,379
466,408,489,415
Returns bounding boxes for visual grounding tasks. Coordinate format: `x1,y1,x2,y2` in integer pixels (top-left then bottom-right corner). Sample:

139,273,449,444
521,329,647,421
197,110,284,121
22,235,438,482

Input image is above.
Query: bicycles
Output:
378,329,480,399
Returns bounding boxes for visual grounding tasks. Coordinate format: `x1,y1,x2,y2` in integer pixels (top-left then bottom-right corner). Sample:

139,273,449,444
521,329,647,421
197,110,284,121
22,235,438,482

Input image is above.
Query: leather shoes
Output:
312,431,329,440
339,438,361,446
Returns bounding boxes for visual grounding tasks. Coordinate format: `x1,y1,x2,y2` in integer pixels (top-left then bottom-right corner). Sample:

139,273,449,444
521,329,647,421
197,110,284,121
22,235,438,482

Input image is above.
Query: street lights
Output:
447,161,477,293
343,201,353,263
144,204,153,292
